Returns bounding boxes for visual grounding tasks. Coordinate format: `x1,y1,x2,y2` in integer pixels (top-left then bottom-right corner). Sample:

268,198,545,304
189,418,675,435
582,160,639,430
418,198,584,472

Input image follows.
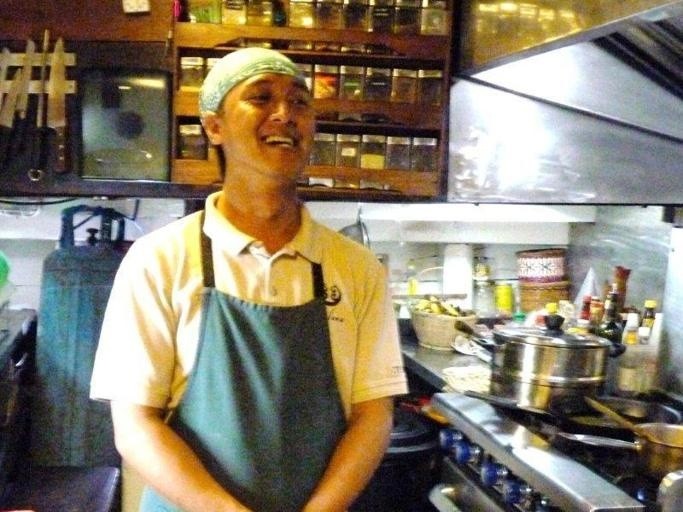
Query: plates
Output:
171,0,455,202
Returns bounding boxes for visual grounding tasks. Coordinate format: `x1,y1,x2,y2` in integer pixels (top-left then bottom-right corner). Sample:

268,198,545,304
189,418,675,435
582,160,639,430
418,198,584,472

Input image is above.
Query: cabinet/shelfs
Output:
547,264,666,397
474,253,515,316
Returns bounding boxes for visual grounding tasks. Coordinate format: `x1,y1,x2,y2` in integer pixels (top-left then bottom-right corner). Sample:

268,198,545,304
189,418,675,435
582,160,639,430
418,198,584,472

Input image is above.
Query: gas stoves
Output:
428,390,683,512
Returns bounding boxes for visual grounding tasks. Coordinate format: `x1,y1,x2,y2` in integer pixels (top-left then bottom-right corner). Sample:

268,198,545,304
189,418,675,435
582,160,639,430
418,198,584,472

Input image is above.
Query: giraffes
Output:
428,390,683,512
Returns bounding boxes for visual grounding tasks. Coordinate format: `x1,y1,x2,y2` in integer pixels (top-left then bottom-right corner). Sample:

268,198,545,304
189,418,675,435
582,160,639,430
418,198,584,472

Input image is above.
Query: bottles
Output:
182,0,448,39
474,253,515,316
177,49,446,106
547,264,666,397
179,116,437,169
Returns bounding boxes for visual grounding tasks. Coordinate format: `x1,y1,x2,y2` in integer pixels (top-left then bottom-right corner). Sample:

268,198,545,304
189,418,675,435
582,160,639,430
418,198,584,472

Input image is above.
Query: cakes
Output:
495,283,513,312
473,257,489,280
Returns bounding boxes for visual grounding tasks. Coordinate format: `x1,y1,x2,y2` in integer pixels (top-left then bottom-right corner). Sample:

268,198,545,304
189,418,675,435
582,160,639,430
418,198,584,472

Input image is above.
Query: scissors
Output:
33,29,58,173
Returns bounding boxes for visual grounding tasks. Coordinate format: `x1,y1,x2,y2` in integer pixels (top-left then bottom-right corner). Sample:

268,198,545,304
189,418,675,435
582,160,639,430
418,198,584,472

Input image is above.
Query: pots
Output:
454,315,681,481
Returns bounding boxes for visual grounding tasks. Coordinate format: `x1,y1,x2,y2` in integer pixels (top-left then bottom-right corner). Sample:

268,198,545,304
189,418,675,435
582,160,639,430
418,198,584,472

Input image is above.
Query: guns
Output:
416,295,460,316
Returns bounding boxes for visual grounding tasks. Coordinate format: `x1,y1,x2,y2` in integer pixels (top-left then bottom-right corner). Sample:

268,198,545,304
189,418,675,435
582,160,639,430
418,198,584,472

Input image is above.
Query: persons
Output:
89,47,411,512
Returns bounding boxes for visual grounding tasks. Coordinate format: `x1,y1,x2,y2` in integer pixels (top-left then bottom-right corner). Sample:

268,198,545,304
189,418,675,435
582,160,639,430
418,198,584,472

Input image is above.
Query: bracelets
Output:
514,248,574,313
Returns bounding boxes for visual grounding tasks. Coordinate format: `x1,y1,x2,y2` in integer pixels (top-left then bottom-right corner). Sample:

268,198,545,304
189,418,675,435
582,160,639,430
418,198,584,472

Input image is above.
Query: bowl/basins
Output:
514,248,574,313
